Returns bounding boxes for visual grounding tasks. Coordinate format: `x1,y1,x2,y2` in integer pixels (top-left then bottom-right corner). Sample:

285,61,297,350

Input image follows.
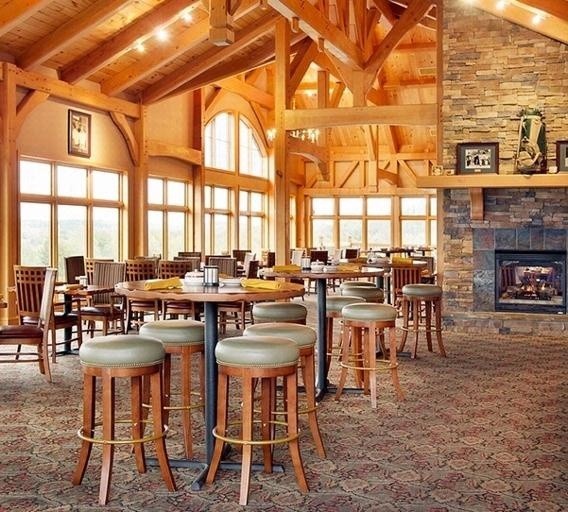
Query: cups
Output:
80,276,88,289
203,266,220,286
301,257,310,271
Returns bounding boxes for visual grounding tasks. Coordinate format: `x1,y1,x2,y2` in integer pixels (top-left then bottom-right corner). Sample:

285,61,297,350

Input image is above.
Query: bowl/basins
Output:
380,248,388,251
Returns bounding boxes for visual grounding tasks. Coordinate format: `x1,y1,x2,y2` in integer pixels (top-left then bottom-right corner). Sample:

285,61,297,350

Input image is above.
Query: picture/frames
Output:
455,142,500,175
556,140,568,173
68,107,92,158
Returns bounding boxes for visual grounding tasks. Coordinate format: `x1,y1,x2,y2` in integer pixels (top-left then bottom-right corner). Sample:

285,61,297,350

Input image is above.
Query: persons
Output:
465,150,490,167
72,121,79,146
78,125,86,151
